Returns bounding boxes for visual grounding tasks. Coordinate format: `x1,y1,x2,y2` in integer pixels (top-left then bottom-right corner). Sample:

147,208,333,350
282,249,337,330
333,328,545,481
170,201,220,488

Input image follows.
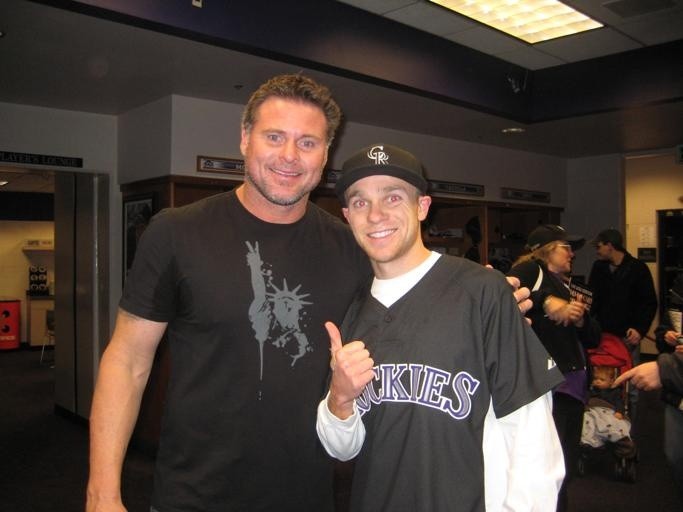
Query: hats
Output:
527,223,586,251
333,143,428,195
591,229,627,243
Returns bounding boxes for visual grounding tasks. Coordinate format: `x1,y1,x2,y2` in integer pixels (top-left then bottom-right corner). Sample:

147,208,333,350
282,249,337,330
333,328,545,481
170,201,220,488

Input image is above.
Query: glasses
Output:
551,243,572,251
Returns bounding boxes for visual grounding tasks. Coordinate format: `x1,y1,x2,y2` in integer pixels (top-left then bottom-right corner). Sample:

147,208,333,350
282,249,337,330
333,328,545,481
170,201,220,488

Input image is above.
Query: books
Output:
567,277,593,328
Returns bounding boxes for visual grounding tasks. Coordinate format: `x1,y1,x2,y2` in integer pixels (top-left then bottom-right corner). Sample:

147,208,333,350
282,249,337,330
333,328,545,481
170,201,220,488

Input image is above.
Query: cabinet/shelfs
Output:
26,290,55,347
122,173,566,286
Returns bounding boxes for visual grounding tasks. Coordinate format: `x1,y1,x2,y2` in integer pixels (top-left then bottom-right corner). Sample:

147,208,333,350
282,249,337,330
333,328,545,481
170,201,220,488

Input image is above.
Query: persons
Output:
504,223,594,512
579,363,636,462
587,226,660,440
314,141,569,512
84,71,535,512
608,353,683,414
652,267,683,474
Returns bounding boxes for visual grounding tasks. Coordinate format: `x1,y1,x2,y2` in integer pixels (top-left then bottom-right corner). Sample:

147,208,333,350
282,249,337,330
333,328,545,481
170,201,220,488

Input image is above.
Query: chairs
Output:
38,309,57,368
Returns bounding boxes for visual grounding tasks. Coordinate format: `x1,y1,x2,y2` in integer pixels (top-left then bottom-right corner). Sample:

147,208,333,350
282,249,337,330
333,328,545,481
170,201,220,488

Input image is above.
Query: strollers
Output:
573,335,635,486
